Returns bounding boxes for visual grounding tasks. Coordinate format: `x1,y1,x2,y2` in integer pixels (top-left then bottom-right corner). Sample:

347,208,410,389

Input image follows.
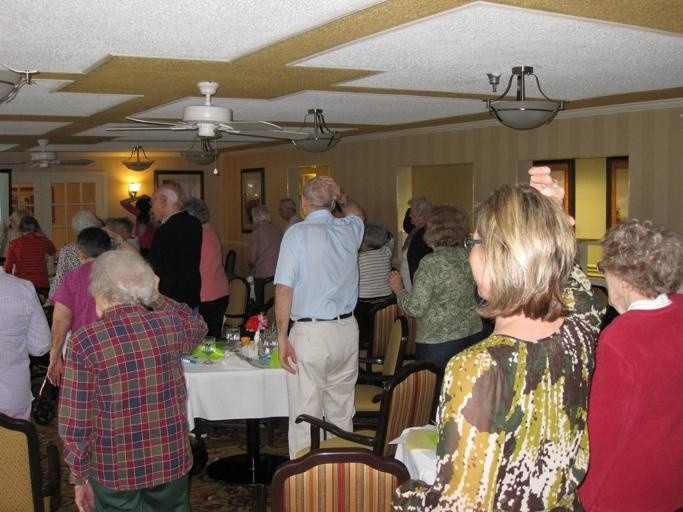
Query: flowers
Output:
244,312,270,332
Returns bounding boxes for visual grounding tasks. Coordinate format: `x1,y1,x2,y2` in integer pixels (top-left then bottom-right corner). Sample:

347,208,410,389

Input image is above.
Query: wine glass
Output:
224,325,241,351
200,336,216,364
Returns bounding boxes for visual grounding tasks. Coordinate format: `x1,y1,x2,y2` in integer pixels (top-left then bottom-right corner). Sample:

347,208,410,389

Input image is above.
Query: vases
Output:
606,157,629,230
533,160,575,232
254,330,260,345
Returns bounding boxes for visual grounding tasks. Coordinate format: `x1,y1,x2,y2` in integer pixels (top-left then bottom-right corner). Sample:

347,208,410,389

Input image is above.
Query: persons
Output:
358,223,394,342
111,183,230,340
1,220,52,420
273,176,365,460
391,168,609,511
248,199,304,313
45,210,141,399
578,217,683,511
58,250,208,512
1,210,56,306
388,198,485,370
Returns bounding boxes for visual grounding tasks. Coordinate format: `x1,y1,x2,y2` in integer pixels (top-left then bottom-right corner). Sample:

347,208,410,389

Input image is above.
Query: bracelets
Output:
339,195,350,206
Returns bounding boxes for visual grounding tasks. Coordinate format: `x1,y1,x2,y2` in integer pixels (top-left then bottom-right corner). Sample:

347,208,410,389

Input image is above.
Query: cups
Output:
241,337,250,346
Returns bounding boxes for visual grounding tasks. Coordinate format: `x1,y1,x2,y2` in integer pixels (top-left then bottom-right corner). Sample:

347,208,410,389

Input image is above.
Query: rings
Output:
552,175,557,186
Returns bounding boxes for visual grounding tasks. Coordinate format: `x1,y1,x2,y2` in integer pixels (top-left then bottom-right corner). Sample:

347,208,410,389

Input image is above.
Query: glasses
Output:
464,236,481,249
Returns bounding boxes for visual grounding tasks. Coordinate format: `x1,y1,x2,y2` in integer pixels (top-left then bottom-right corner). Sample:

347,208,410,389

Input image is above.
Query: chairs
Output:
224,277,250,328
0,412,60,512
360,297,416,375
270,448,410,512
295,361,444,463
260,275,275,307
354,315,409,431
264,297,276,327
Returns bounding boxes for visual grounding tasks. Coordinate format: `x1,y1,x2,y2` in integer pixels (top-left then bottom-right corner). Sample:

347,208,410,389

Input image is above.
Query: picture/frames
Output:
240,167,267,233
154,170,204,202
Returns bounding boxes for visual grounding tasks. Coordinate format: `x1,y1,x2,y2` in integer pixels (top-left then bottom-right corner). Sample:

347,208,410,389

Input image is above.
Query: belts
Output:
297,312,351,322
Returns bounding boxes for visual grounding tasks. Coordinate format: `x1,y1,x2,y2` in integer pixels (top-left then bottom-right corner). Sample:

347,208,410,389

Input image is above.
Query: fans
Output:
0,139,95,168
106,81,309,140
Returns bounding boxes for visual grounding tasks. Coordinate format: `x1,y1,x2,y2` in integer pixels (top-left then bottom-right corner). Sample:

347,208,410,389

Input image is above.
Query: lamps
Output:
186,135,219,165
291,109,342,152
129,183,141,198
486,66,565,129
123,145,154,170
0,61,38,104
